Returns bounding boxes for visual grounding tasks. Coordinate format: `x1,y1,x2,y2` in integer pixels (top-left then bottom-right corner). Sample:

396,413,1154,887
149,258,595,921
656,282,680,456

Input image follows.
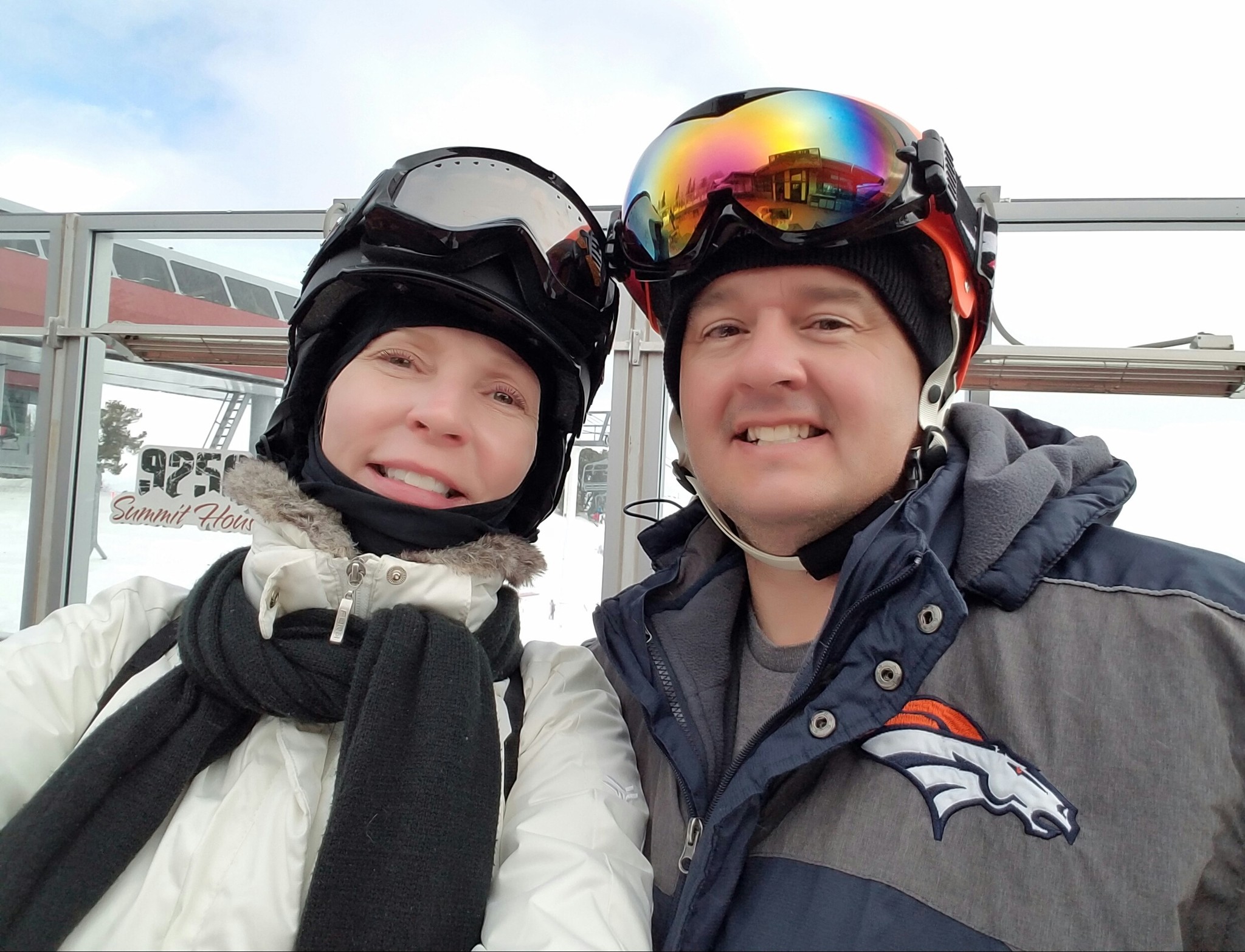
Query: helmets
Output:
284,209,592,450
621,89,999,332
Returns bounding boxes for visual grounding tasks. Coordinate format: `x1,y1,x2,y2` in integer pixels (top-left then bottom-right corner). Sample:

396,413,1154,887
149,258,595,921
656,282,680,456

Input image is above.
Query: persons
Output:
0,142,657,952
574,84,1245,951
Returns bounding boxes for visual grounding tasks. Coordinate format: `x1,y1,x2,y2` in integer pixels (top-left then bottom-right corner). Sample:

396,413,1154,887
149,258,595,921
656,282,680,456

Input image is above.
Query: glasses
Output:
367,146,614,322
620,89,914,278
